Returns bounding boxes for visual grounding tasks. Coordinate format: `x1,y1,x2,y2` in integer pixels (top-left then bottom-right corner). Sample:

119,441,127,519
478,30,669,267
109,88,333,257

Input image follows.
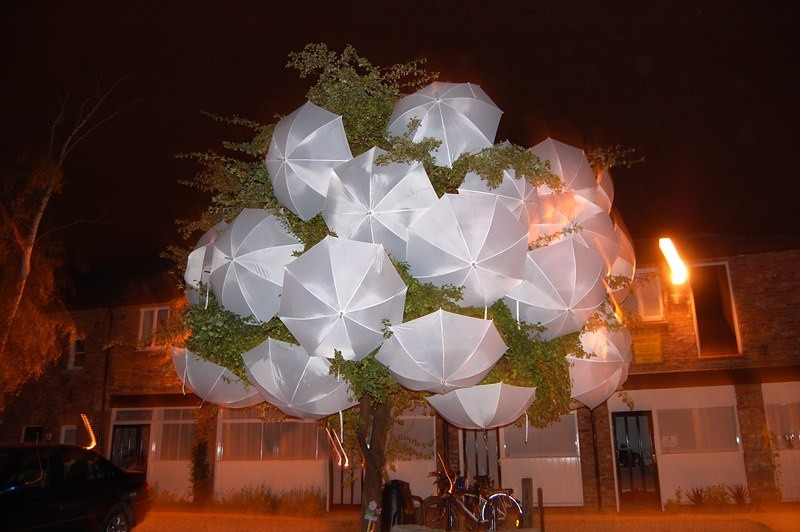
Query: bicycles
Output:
417,469,525,532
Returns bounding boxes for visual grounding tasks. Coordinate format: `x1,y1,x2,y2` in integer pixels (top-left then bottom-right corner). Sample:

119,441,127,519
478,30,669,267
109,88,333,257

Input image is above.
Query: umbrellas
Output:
168,82,637,440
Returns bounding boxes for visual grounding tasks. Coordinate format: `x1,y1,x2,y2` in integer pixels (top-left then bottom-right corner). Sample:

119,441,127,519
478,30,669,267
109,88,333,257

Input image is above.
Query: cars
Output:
0,442,151,532
